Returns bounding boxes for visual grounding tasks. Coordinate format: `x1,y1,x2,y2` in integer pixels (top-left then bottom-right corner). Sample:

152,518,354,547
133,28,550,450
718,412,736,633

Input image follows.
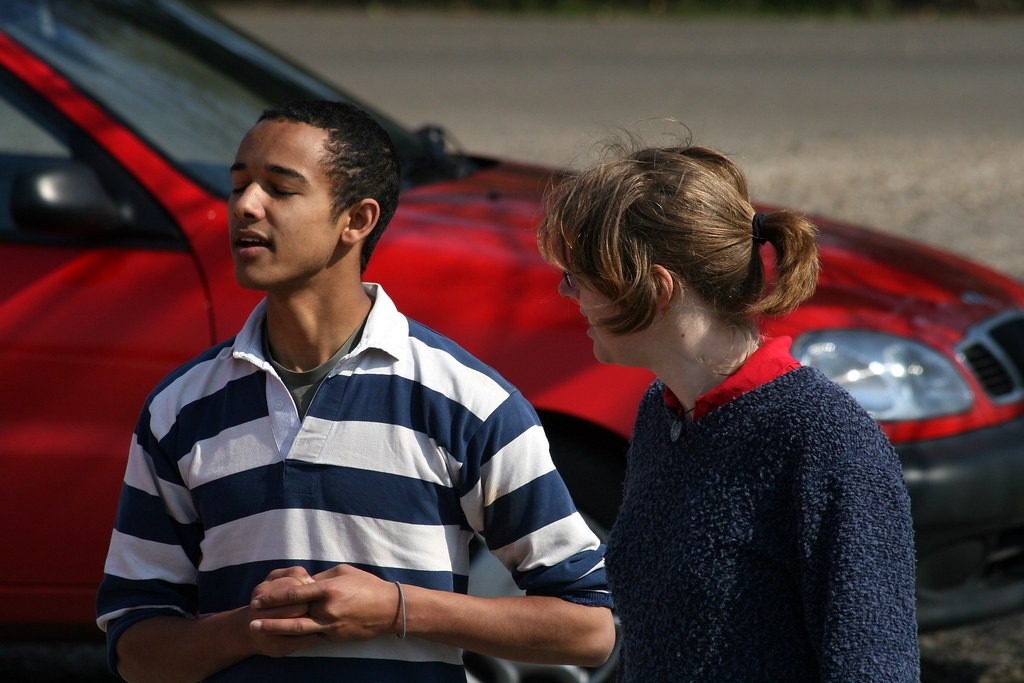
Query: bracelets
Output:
396,579,406,638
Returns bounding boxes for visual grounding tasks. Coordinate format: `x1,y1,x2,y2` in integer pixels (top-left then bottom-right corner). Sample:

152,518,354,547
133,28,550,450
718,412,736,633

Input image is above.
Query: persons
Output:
96,101,616,683
538,148,920,683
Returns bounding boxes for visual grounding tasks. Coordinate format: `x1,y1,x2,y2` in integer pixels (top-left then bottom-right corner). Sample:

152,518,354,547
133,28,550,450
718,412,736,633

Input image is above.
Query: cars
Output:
0,0,1024,669
